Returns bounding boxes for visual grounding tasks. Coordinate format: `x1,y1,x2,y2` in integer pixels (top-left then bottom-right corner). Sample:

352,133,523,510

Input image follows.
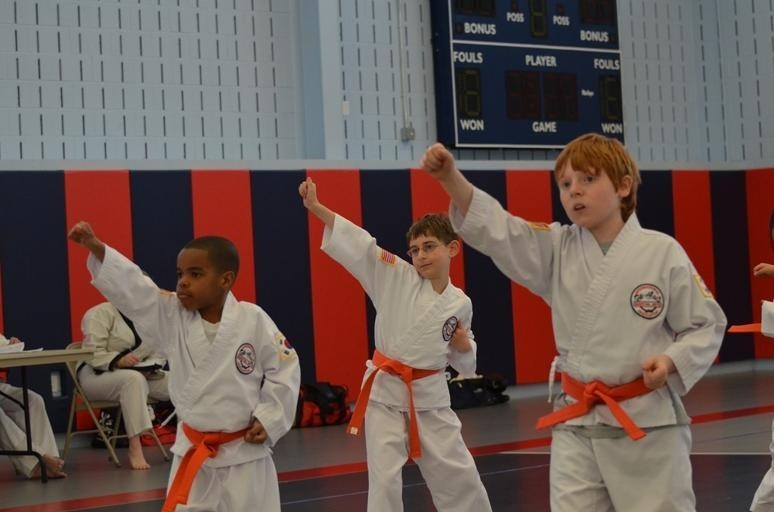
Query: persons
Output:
417,132,727,511
747,212,773,511
75,301,171,470
0,332,69,481
297,177,494,511
67,219,304,511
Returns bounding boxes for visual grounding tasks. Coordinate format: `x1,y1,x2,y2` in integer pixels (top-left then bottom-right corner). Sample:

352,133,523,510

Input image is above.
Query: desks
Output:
0,349,122,484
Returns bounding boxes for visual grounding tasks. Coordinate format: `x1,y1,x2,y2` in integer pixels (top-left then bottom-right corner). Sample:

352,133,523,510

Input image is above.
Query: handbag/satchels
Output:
446,366,517,409
290,381,355,429
90,399,177,452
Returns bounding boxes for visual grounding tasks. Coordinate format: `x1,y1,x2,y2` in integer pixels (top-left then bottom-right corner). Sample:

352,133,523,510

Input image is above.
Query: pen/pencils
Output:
128,347,134,353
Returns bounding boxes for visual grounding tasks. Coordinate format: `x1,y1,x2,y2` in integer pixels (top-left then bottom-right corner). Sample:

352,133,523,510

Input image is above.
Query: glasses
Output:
407,243,448,258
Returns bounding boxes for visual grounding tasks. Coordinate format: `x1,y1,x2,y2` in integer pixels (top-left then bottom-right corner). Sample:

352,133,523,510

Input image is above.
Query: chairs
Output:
62,340,170,467
